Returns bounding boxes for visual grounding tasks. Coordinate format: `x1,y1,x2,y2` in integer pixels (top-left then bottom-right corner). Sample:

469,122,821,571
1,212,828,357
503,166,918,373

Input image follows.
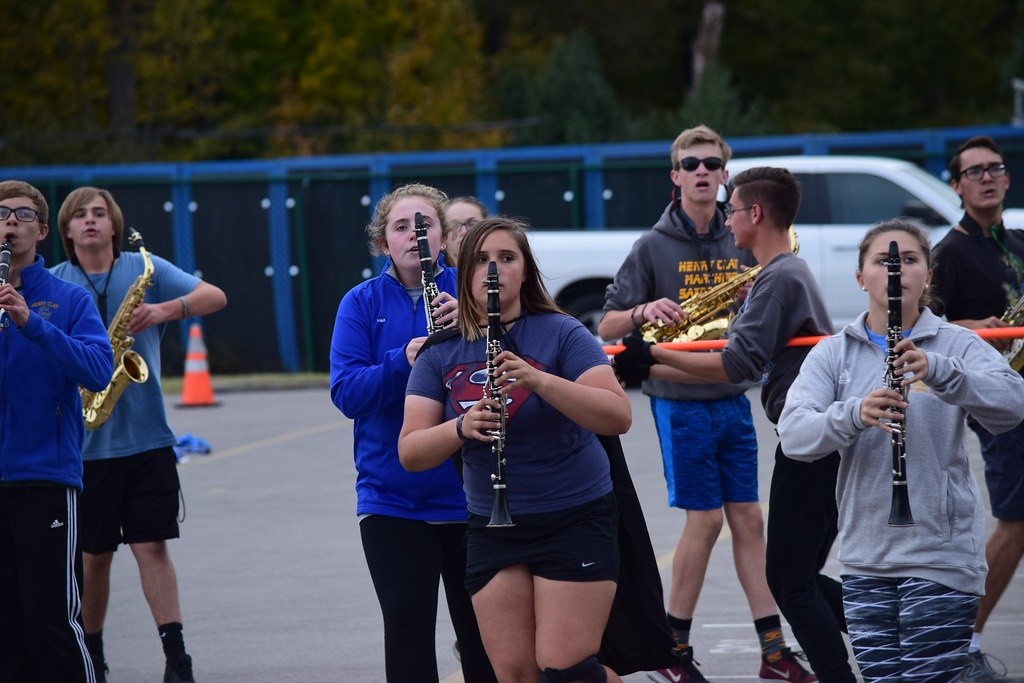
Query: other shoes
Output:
960,649,1016,683
93,664,109,683
163,654,195,683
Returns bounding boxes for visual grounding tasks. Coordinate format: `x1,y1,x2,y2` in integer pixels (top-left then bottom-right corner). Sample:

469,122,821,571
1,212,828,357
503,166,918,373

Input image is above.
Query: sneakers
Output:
647,646,710,683
759,647,820,683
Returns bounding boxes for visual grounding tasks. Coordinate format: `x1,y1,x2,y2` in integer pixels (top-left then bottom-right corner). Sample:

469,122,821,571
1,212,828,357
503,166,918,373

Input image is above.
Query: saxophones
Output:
412,211,449,336
637,223,802,351
0,231,17,320
977,223,1024,372
75,226,152,432
485,259,516,528
880,239,920,529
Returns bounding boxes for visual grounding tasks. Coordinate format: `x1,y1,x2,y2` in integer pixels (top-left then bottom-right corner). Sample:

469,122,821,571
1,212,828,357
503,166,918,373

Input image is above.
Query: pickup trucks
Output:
524,155,1024,387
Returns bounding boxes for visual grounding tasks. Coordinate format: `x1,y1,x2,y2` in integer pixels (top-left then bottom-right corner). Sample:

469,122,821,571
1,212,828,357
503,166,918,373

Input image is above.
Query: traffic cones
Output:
173,323,223,409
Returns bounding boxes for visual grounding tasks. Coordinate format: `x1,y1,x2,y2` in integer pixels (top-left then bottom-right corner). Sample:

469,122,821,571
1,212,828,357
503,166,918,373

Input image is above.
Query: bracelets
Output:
641,302,648,323
179,296,190,319
456,414,467,441
630,305,639,327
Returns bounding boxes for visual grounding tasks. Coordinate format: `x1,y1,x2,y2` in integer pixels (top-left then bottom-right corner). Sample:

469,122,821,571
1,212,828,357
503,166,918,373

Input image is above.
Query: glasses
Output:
953,163,1008,182
724,203,753,218
447,221,479,233
0,205,42,222
676,156,725,171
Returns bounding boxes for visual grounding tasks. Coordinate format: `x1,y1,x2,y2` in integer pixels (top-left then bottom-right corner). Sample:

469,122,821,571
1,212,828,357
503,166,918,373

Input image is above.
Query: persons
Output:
597,124,819,683
43,187,226,683
330,184,706,683
922,136,1024,683
614,166,857,683
775,221,1024,683
0,179,116,683
